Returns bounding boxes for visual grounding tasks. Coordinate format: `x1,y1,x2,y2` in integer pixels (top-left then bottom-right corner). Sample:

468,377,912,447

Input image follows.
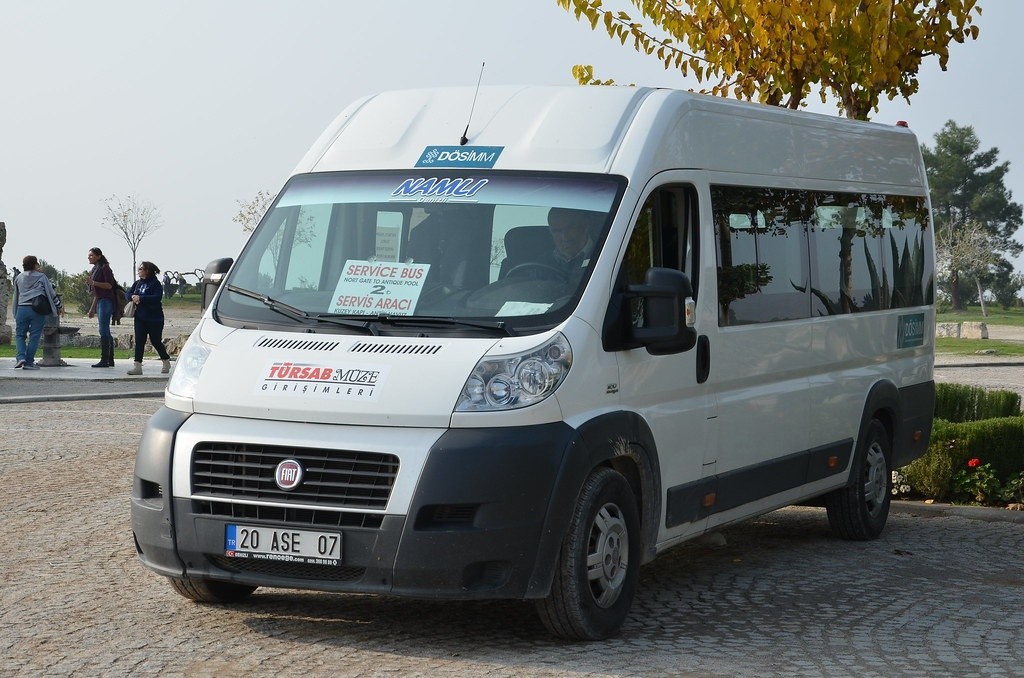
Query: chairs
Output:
498,226,556,280
619,210,654,288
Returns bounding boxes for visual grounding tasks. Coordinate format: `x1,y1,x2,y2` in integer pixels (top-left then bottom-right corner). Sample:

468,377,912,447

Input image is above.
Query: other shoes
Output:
23,363,40,370
15,359,27,368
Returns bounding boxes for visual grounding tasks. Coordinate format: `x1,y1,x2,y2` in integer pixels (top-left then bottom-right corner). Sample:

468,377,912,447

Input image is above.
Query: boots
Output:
109,335,115,366
92,335,110,367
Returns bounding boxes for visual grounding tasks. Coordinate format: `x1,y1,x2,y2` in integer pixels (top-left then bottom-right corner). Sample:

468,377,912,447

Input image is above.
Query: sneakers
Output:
127,361,143,375
161,359,171,373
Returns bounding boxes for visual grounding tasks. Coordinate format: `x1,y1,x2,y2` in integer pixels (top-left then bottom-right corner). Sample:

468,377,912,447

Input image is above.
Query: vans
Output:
130,83,937,641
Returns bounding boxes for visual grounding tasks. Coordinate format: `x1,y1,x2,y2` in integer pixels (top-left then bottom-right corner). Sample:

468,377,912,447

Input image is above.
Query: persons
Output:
11,256,65,370
528,207,598,291
87,247,117,367
124,262,170,374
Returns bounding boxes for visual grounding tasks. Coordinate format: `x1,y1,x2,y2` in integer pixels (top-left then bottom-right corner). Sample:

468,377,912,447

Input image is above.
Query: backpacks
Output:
111,281,129,325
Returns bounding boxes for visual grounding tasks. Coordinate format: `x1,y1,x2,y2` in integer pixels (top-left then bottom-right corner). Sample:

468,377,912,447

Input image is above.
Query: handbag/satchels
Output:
31,293,52,315
123,279,141,318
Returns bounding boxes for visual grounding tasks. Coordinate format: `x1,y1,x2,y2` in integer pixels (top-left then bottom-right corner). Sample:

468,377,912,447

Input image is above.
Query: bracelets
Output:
92,280,95,285
